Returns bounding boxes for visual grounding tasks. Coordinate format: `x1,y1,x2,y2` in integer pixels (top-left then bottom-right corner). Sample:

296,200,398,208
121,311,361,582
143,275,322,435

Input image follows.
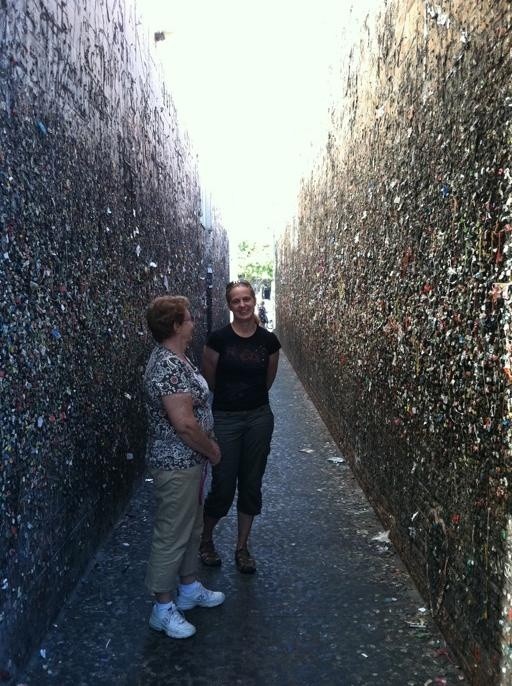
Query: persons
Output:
201,280,282,573
260,302,269,329
141,294,227,639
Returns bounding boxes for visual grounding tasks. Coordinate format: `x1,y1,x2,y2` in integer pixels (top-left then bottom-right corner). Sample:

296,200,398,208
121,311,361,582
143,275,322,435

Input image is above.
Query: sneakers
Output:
200,540,221,566
235,549,255,574
148,600,196,639
175,580,226,611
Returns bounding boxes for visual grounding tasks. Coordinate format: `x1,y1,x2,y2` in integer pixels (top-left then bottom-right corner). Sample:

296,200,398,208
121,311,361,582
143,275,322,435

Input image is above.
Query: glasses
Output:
183,316,195,323
226,280,249,289
